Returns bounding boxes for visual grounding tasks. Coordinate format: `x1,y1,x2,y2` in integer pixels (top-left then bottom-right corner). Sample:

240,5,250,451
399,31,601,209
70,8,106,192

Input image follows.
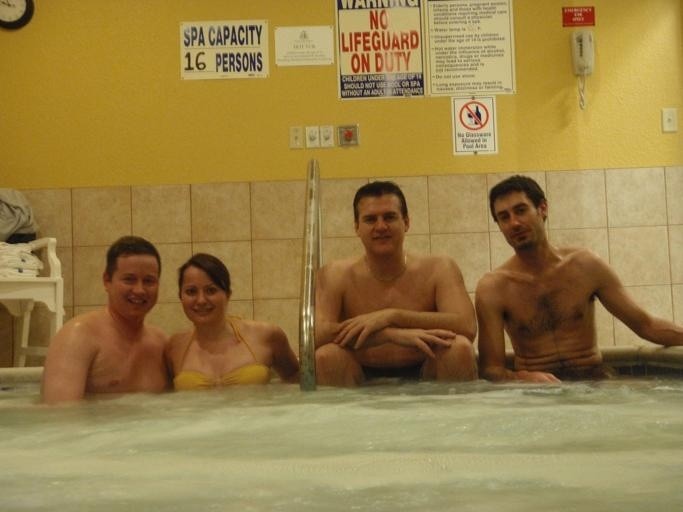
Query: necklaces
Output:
363,250,408,283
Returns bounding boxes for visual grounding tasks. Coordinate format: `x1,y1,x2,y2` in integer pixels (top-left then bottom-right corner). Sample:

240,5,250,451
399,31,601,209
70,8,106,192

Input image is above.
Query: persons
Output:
40,235,169,404
315,180,478,388
475,175,683,384
163,252,299,393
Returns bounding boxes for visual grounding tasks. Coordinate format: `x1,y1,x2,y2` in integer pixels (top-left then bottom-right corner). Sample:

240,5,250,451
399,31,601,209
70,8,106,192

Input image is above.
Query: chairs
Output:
1,187,65,369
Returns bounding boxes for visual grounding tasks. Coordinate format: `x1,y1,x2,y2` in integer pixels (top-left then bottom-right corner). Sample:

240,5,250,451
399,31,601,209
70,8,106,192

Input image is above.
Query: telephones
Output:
572,29,595,78
